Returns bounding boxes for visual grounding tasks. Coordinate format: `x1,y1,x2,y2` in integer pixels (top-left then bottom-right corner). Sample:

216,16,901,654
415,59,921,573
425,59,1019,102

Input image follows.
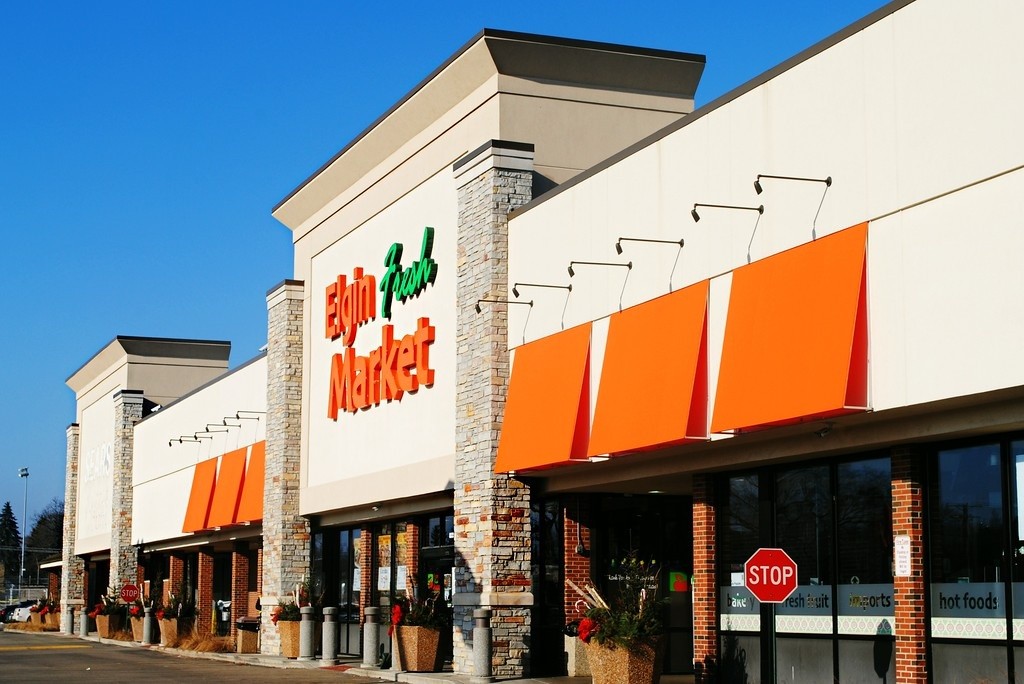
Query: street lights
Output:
18,467,29,584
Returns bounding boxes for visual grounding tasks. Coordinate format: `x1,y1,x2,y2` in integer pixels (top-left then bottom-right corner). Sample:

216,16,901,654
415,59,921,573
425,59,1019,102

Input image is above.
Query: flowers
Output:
380,591,451,630
155,592,183,620
89,593,121,617
128,599,152,620
577,566,659,657
30,598,61,615
270,582,317,625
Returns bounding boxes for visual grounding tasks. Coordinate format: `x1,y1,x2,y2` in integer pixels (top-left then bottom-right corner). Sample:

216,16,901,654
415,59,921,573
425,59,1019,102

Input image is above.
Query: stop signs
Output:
744,549,798,603
120,584,139,602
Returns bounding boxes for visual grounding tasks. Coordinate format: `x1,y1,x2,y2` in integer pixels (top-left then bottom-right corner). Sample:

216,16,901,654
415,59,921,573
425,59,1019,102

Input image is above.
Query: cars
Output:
0,599,42,623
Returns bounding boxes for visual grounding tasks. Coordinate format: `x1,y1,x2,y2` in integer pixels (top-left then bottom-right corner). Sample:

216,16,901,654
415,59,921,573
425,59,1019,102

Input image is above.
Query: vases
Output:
274,621,301,658
393,625,441,672
95,614,113,638
158,617,181,646
129,615,144,642
30,611,61,625
584,637,658,684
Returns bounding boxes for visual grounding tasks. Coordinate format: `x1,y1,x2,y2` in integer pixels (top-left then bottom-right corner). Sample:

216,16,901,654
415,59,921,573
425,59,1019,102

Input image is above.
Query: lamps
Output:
754,174,832,195
194,429,228,440
512,283,572,298
236,411,266,419
222,416,259,425
568,261,632,278
179,436,212,443
616,238,684,254
475,299,533,313
691,203,763,222
205,423,241,432
169,439,201,446
